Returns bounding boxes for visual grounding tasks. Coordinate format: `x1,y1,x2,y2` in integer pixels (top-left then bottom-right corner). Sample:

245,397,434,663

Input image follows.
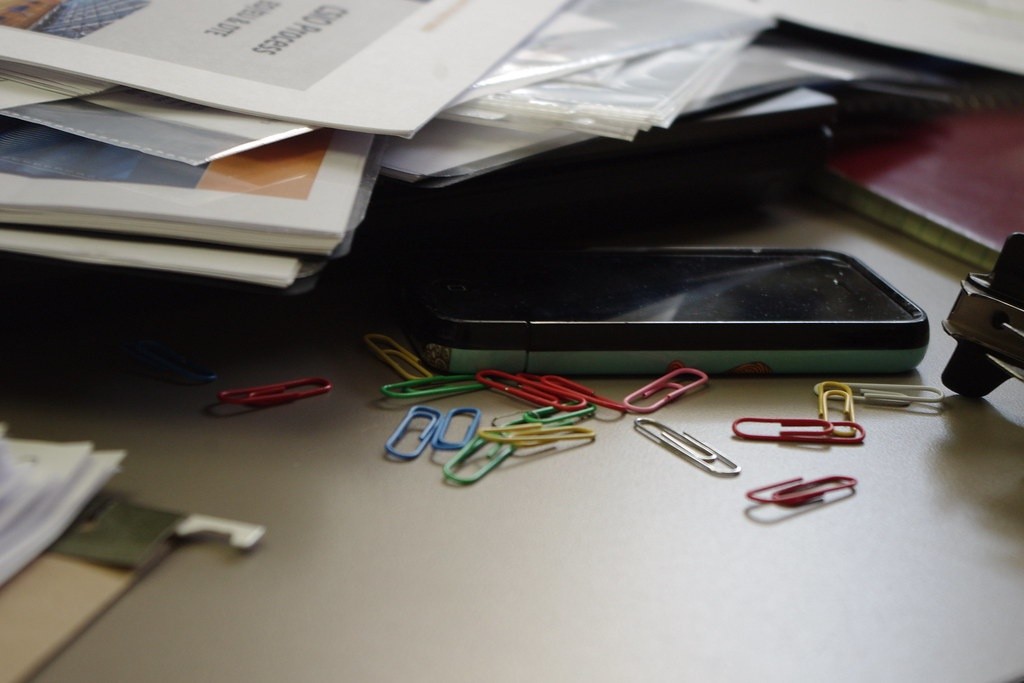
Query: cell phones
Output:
398,245,930,376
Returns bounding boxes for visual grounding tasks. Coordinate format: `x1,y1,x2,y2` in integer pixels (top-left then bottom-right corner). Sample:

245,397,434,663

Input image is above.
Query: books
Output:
0,0,1024,286
